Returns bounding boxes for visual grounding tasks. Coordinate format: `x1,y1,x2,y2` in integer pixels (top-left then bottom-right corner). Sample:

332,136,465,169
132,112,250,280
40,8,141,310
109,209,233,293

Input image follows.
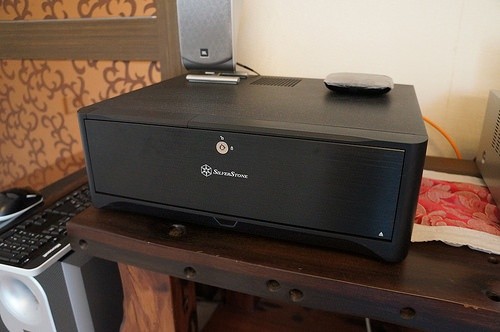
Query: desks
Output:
66,155,500,332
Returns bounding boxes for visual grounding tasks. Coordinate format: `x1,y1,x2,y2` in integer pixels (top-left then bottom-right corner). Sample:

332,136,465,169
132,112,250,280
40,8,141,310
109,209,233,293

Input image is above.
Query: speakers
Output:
0,248,126,332
176,0,240,84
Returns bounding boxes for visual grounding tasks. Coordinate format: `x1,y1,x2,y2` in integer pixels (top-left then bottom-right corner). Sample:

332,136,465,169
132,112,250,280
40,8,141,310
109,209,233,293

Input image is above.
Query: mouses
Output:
0,187,44,222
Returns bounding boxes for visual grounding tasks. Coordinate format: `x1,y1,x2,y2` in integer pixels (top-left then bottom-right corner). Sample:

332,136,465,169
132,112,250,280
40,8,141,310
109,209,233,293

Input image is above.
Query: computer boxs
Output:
77,69,428,265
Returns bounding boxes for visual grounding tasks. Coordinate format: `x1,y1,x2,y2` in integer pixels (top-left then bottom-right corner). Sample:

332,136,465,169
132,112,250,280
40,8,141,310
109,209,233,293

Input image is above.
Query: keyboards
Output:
0,184,94,276
